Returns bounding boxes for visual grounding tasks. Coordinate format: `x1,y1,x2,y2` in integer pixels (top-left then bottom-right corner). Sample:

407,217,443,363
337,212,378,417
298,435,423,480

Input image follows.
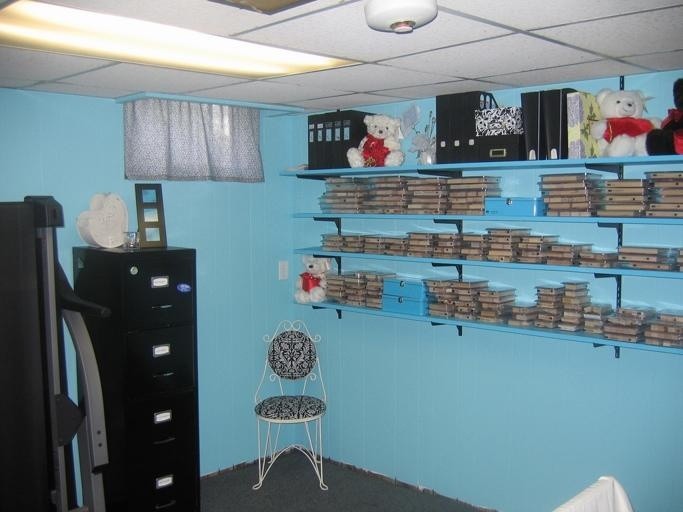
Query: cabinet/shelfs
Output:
73,246,202,512
277,153,683,358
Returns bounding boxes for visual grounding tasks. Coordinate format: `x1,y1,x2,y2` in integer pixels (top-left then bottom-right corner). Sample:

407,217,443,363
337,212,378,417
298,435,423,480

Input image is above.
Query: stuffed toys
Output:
591,87,660,156
346,113,404,168
646,78,682,156
293,255,332,303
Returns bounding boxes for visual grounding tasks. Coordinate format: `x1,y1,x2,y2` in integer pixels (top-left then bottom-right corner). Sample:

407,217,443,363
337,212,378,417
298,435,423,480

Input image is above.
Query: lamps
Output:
364,1,438,34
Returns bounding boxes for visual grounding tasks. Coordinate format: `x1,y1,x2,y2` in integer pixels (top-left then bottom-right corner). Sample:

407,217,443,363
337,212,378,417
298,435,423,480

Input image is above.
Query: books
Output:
327,274,394,309
319,228,683,273
539,171,683,218
420,274,683,348
320,176,501,217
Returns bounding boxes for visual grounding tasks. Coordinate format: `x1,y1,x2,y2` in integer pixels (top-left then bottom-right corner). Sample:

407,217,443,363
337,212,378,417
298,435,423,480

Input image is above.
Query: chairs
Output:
252,319,331,490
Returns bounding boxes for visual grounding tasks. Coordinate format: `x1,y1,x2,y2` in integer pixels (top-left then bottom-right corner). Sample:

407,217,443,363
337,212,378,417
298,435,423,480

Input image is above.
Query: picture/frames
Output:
135,183,168,248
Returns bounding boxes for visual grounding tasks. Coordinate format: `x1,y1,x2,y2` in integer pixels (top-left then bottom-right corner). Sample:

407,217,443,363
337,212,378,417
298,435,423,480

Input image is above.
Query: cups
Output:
120,231,140,250
